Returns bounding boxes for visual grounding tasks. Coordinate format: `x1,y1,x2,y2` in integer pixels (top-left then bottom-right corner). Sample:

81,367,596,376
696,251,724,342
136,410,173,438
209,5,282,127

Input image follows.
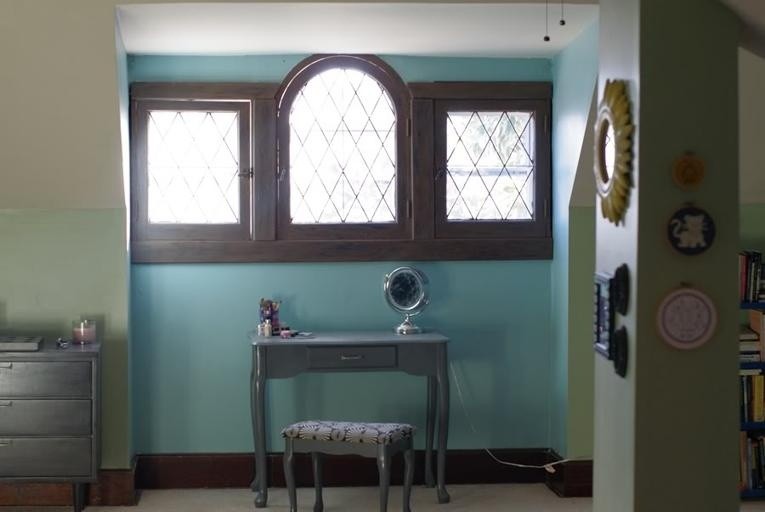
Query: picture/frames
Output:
590,271,614,359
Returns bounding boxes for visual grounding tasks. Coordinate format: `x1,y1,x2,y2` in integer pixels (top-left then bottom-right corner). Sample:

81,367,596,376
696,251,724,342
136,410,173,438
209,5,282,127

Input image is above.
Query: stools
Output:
281,419,416,512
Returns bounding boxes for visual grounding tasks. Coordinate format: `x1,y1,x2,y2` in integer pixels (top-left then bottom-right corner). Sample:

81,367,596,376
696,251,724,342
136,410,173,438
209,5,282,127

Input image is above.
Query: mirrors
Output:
592,78,632,224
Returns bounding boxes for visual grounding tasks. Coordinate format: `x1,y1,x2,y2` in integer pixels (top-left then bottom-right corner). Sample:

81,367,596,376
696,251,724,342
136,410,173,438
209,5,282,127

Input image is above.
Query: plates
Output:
670,153,706,191
667,206,716,256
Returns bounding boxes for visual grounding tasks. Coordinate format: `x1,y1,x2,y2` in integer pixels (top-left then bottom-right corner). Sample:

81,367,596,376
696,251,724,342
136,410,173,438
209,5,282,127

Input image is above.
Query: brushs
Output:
260,298,282,314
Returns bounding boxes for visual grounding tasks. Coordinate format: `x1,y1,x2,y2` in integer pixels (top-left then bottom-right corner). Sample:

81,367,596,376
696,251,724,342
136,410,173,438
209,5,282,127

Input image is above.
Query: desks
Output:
250,329,450,507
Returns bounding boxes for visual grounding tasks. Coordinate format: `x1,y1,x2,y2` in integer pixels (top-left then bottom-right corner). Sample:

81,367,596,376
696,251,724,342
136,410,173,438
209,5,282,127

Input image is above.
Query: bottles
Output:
257,308,281,338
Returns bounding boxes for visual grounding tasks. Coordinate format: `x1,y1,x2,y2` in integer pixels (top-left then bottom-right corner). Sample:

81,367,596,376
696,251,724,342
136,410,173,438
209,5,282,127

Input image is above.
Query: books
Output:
738,251,764,498
0,336,43,352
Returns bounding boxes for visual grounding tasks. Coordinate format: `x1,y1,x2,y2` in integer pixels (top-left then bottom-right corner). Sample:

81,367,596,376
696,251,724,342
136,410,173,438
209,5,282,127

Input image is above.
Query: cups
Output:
71,318,96,344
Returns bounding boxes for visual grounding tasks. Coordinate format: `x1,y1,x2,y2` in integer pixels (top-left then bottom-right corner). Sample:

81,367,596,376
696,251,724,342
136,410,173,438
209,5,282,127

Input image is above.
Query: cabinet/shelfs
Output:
737,299,765,499
0,361,93,479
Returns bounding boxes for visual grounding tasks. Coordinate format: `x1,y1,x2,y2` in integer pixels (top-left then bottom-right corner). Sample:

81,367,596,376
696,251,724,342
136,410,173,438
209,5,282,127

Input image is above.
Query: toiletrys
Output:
257,319,291,340
